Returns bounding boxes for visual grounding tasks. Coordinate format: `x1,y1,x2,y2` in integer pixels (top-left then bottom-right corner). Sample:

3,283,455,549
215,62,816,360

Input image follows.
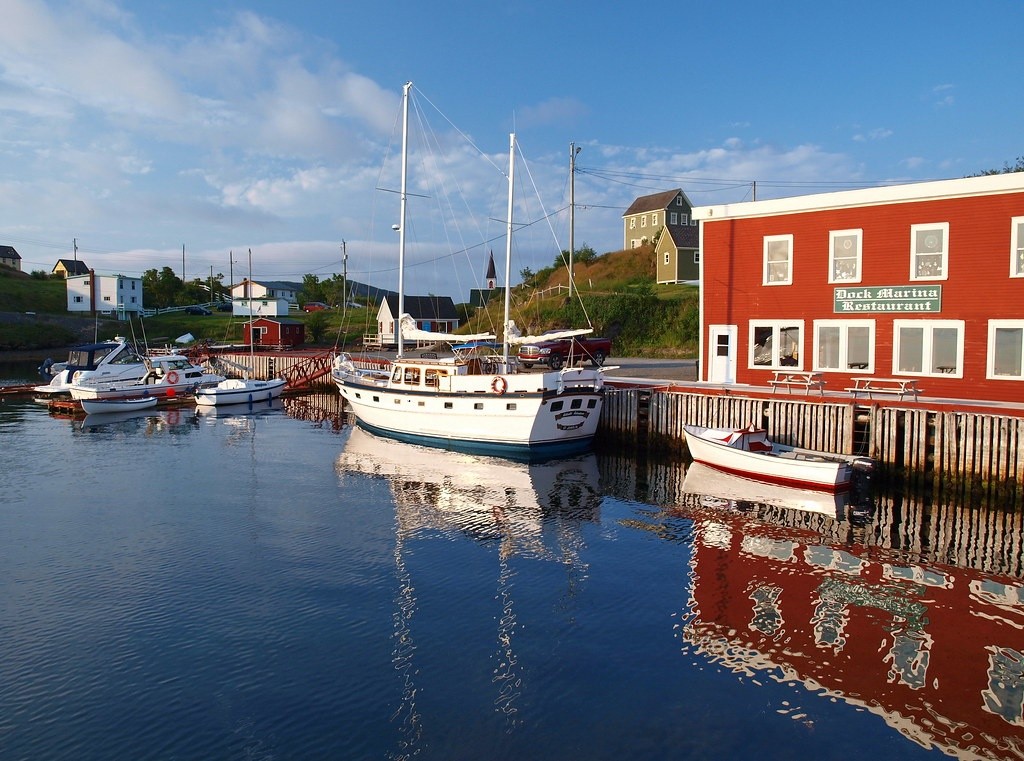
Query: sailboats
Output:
333,425,604,761
332,81,619,453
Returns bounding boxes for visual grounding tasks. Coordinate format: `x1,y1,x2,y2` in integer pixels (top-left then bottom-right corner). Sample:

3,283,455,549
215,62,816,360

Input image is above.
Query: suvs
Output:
217,302,233,312
185,305,213,316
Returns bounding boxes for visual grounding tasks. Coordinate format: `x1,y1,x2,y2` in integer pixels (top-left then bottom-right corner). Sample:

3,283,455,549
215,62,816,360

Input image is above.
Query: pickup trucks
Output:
516,329,611,370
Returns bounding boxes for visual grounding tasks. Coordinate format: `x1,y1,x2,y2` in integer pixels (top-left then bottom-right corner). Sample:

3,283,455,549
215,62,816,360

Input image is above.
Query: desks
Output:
772,371,823,396
937,365,956,373
848,362,868,369
850,377,918,402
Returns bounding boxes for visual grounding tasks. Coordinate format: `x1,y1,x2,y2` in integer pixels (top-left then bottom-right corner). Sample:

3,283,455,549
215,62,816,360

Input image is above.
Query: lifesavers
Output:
490,375,508,396
167,371,180,385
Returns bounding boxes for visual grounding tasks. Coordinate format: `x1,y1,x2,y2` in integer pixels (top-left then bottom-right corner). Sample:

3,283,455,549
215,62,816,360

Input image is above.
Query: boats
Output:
31,313,226,406
194,398,285,427
192,367,287,406
81,407,161,429
81,396,158,415
683,422,853,490
682,461,845,521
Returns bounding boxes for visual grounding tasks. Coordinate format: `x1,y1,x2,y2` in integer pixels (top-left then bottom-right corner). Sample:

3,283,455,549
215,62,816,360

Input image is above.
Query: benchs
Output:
784,378,828,384
844,388,908,394
862,387,925,394
767,380,813,385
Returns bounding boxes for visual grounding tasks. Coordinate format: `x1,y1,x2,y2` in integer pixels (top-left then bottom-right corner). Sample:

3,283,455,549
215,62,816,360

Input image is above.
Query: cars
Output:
302,302,326,312
